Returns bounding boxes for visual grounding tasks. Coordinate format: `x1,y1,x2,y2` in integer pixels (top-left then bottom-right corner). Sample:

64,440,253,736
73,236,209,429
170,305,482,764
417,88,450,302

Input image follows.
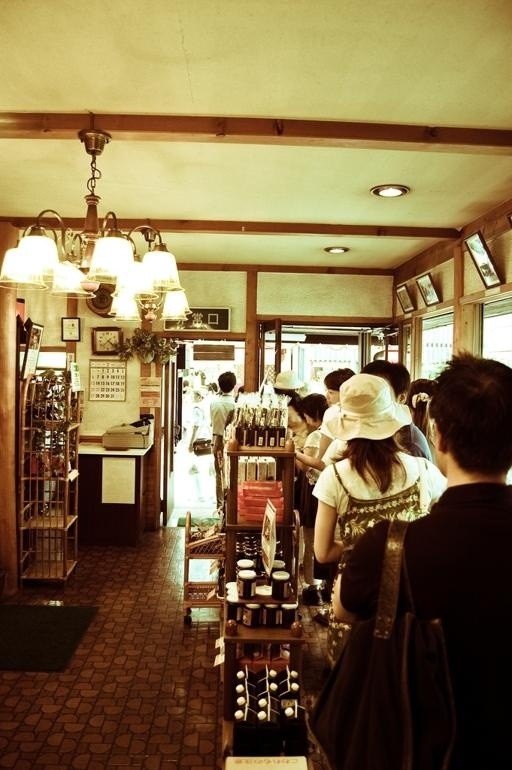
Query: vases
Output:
137,349,155,364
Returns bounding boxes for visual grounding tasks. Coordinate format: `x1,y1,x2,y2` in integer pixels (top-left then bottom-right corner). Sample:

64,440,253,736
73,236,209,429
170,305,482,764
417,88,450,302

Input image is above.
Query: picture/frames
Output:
20,322,44,381
395,284,417,314
463,229,505,290
61,317,80,341
507,212,512,224
415,272,442,306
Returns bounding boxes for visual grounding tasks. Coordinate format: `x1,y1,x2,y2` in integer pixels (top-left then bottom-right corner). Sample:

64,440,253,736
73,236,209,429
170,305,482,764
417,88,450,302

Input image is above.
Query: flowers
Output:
119,327,180,361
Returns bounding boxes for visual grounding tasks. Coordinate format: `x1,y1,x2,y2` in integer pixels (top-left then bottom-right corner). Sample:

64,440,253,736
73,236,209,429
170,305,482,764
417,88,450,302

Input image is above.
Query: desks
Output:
76,441,154,546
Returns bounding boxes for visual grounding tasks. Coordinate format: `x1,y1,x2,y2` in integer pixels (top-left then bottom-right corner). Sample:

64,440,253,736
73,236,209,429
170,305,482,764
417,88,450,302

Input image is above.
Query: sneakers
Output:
301,583,332,606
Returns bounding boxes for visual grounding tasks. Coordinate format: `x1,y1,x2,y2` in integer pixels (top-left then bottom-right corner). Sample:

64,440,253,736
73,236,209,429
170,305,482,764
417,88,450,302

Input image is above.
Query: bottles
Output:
233,642,307,756
232,404,288,450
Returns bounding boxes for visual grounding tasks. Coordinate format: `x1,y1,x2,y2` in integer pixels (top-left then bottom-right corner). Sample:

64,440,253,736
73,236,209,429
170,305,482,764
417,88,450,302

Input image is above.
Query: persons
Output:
188,370,334,606
329,347,511,770
310,360,448,663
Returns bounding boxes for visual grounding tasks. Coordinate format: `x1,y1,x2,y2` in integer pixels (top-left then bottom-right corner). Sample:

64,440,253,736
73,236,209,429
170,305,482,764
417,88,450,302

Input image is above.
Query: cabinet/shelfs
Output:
218,438,308,770
19,373,81,588
182,512,225,630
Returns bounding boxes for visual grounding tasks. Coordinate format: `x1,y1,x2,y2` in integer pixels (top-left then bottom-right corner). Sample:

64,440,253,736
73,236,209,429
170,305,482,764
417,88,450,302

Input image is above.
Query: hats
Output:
273,370,305,391
321,372,414,443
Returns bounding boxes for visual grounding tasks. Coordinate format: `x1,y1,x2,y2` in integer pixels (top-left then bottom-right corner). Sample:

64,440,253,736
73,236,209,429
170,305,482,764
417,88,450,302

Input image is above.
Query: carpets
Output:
0,603,100,672
177,516,220,527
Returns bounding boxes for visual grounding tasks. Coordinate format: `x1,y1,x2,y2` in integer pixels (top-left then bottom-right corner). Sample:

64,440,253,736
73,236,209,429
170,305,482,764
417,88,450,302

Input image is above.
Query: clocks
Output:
91,326,123,355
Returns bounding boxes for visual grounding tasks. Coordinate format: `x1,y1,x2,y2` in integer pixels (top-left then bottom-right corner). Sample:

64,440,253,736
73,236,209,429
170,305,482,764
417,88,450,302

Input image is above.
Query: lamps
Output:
0,128,193,324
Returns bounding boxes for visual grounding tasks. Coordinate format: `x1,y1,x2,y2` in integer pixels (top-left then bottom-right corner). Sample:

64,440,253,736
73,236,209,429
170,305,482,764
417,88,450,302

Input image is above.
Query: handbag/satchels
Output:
305,519,452,770
